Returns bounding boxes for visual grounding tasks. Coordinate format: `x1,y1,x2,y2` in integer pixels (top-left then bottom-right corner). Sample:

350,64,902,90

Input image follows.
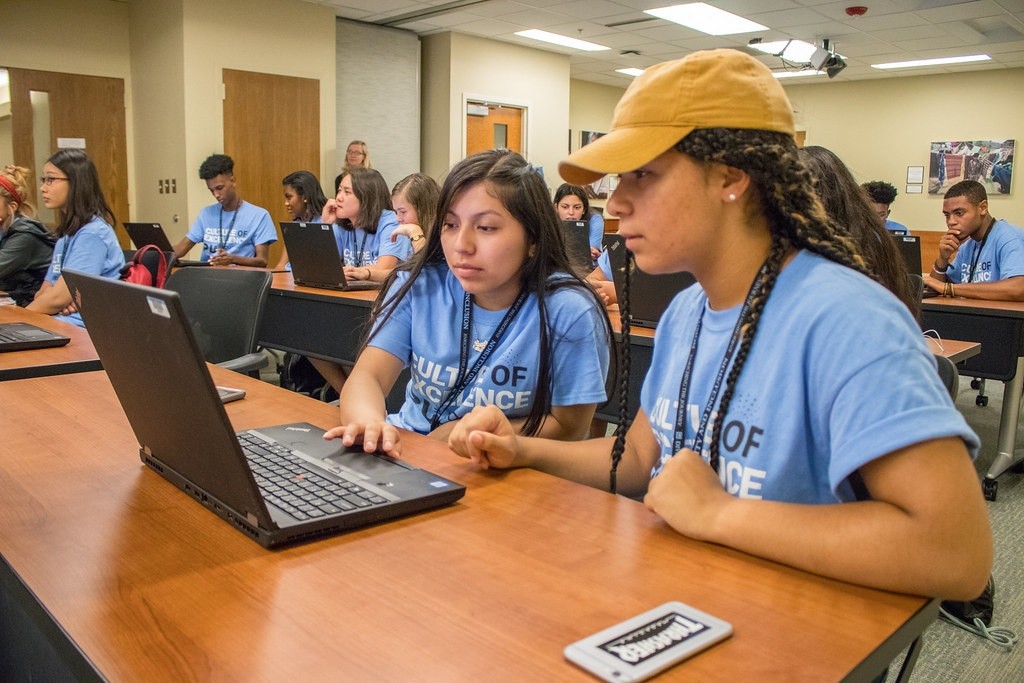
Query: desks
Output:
0,263,1024,683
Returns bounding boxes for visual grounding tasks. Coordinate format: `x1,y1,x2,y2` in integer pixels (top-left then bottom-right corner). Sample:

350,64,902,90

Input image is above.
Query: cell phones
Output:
564,601,734,683
215,385,245,403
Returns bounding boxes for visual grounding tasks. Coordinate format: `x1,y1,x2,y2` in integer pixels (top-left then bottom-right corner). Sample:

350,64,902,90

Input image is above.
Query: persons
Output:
321,148,618,456
172,153,277,267
864,181,910,236
968,154,982,183
278,171,327,269
555,181,606,263
583,250,616,305
335,141,373,196
307,168,415,404
391,174,442,252
449,49,995,599
800,145,917,316
0,165,60,306
25,147,125,328
923,180,1024,301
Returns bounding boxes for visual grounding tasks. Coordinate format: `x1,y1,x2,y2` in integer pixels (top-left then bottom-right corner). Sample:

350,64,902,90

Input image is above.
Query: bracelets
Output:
943,283,954,298
933,259,955,274
365,269,371,280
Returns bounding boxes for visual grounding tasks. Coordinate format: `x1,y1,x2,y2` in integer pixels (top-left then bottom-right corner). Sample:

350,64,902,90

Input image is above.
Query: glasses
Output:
40,176,69,185
347,149,363,156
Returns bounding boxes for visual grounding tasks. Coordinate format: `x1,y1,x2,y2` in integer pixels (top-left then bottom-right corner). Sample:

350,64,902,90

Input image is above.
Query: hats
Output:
560,50,798,185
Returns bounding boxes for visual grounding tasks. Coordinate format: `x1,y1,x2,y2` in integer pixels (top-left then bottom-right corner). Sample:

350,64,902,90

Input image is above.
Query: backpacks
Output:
120,244,167,288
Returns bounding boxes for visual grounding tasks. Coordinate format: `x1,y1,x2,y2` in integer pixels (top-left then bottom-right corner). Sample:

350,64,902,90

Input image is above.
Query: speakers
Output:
810,47,832,71
827,55,847,78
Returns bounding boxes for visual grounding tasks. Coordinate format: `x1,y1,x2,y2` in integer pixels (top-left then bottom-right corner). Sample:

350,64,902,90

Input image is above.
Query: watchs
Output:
411,234,425,242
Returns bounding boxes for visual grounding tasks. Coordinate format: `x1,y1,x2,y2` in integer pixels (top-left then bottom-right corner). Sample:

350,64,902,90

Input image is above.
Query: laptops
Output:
61,268,466,550
605,233,699,329
279,221,386,292
561,219,596,273
0,321,71,353
122,222,213,267
887,229,941,299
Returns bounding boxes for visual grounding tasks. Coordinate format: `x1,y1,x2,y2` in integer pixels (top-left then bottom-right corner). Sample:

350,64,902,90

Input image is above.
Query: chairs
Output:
122,250,158,289
163,267,272,380
